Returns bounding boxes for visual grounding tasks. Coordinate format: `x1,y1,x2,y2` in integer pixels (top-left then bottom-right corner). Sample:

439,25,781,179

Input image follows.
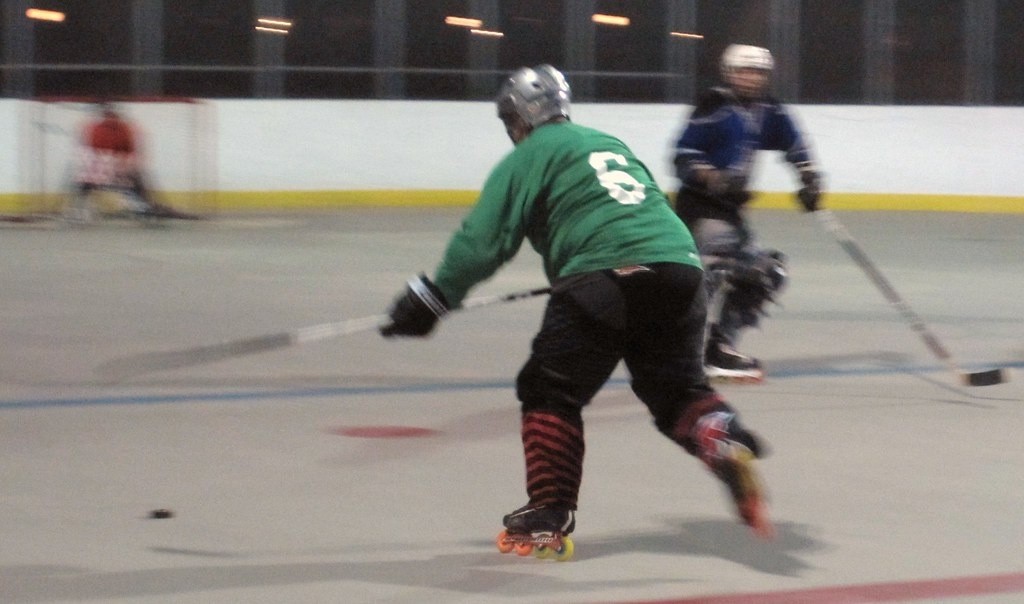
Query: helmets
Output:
719,43,775,70
497,63,571,127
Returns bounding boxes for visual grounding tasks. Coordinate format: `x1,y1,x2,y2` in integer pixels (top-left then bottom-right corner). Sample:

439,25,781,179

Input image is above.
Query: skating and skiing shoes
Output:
702,335,763,385
497,503,576,561
692,412,760,528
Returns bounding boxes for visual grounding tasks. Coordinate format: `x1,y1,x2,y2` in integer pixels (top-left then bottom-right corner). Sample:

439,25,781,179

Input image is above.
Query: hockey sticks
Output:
797,182,1010,387
94,282,554,383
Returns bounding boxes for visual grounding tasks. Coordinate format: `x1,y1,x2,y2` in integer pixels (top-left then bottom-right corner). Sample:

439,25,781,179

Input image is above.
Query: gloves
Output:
705,172,751,207
379,272,450,338
798,171,822,213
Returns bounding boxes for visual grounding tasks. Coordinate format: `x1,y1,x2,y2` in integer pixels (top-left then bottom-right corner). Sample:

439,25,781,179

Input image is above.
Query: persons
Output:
377,64,777,561
64,101,159,222
673,43,826,384
